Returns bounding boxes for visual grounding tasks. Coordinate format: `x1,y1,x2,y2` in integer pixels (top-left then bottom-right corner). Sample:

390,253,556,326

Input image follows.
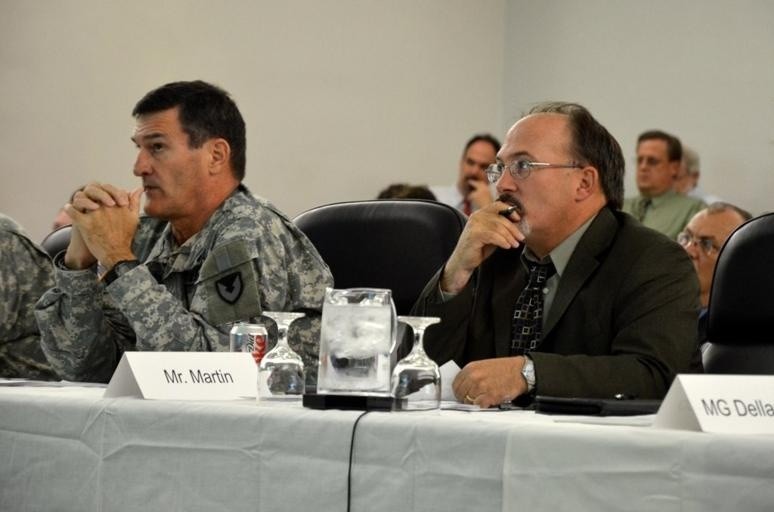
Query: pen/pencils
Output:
497,205,518,217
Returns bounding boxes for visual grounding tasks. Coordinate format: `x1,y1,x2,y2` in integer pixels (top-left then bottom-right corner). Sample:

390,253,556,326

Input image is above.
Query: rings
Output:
465,394,475,403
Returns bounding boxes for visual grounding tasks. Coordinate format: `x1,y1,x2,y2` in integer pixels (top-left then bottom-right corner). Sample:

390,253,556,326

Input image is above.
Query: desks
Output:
0,378,774,512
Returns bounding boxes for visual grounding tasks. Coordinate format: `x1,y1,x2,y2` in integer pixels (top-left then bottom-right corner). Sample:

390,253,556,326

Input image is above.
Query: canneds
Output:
229,321,269,368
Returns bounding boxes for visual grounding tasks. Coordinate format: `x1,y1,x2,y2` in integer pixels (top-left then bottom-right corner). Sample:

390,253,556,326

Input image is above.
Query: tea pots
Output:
316,286,397,402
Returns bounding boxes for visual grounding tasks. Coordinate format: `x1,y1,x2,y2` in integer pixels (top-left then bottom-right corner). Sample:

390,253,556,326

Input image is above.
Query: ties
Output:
462,198,472,218
510,257,548,356
635,196,652,224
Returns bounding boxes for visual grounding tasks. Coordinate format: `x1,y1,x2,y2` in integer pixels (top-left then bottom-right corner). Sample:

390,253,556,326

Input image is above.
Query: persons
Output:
621,129,706,243
425,132,501,219
675,199,754,376
50,186,86,230
671,147,721,206
376,178,440,205
31,79,336,397
392,101,706,410
0,211,62,382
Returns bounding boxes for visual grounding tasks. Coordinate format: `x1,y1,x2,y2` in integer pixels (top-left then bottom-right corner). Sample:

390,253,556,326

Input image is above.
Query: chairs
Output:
293,198,477,316
700,212,774,374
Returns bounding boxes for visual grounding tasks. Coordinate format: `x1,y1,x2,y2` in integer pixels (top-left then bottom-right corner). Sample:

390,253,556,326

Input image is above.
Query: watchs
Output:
521,354,538,393
100,256,141,289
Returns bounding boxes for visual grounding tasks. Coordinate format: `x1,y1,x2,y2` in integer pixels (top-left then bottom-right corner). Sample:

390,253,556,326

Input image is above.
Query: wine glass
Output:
394,312,443,412
256,308,306,401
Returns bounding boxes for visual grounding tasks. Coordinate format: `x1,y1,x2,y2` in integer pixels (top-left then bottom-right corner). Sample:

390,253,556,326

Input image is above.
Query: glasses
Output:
675,228,724,258
482,159,579,185
